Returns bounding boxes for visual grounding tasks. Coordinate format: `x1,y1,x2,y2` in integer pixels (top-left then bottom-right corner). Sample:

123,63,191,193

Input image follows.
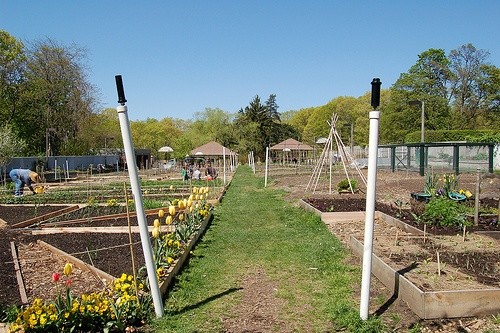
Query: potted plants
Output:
337,178,359,196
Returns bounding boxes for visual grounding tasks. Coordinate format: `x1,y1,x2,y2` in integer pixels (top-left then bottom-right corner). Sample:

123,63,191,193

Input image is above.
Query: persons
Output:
204,164,217,179
9,169,39,198
181,162,201,180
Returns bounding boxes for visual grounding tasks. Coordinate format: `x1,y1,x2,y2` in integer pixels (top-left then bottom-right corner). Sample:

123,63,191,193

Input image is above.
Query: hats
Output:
29,172,38,182
